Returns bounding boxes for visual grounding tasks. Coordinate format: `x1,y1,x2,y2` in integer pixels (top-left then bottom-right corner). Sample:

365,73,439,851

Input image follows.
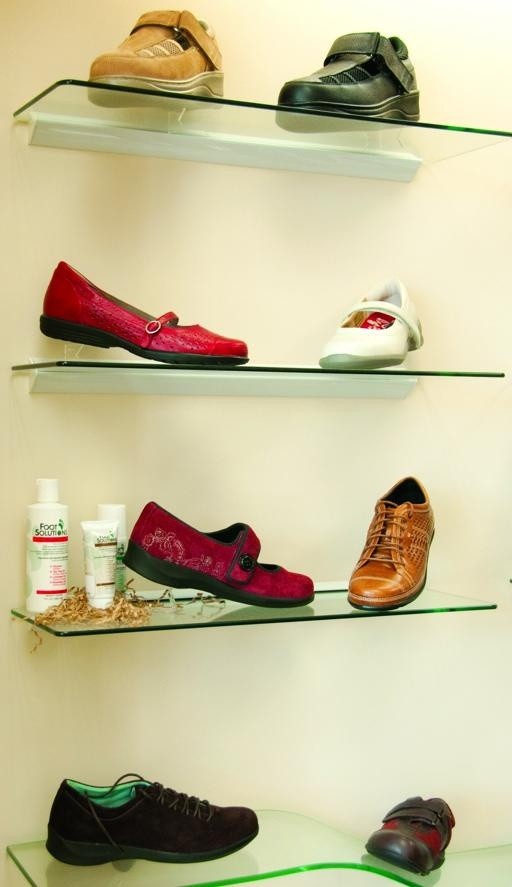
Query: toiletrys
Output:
96,502,126,591
27,478,69,611
80,519,118,609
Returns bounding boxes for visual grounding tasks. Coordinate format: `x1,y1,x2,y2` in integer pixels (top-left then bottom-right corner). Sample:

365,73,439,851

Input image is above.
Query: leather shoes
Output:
364,795,456,877
317,278,426,371
37,259,250,368
346,475,438,615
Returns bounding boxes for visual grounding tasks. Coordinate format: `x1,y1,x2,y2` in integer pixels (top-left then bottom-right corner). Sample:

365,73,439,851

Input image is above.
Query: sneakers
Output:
87,6,228,115
117,498,316,610
42,769,262,869
273,28,422,136
